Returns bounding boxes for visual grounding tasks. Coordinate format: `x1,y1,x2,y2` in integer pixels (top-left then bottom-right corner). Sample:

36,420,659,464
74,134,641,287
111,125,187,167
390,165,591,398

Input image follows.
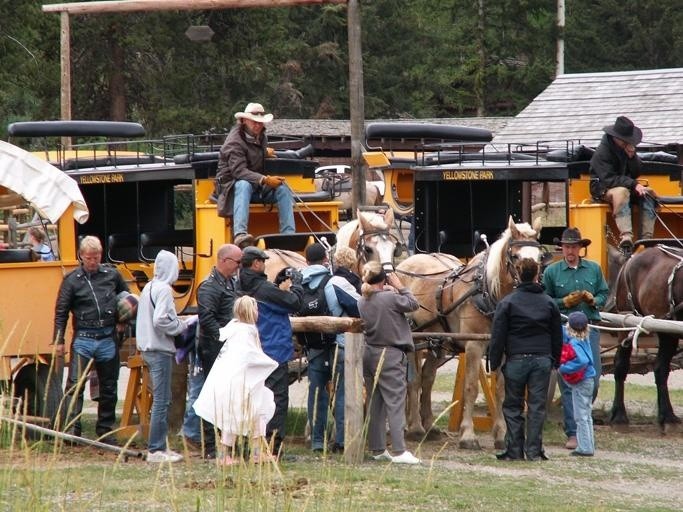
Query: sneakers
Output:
252,455,276,463
494,452,525,461
619,234,635,249
146,451,180,463
216,455,241,466
232,234,255,249
370,448,393,461
564,435,578,449
569,451,594,457
331,443,344,454
98,436,121,445
527,451,549,462
164,448,183,460
391,451,423,465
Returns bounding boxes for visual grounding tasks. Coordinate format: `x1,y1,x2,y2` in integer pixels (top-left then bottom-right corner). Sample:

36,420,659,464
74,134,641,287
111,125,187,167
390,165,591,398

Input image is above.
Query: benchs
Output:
358,120,495,215
566,159,682,244
106,230,151,289
137,229,194,287
5,118,173,172
189,156,343,232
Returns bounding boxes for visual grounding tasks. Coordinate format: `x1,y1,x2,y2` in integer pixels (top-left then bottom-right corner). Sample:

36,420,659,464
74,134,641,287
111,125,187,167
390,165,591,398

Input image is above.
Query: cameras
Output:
286,269,293,277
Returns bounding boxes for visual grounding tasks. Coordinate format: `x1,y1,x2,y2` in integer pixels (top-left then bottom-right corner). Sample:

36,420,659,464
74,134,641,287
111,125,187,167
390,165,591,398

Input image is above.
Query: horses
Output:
313,173,383,221
394,214,544,449
608,245,683,425
261,207,397,443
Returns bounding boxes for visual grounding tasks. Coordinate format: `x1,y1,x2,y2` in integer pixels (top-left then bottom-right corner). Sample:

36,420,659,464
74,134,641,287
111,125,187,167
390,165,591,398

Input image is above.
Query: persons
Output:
541,229,609,448
558,312,595,456
488,258,563,462
589,116,657,251
215,103,295,249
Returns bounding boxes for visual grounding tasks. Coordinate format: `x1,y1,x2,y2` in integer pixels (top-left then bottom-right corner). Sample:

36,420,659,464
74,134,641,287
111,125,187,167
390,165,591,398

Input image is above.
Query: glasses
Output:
246,111,267,116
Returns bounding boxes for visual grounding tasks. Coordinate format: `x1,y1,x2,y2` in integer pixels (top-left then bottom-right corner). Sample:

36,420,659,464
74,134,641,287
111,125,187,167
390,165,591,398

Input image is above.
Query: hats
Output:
554,227,592,247
233,101,274,124
567,311,589,331
602,115,643,145
240,246,270,259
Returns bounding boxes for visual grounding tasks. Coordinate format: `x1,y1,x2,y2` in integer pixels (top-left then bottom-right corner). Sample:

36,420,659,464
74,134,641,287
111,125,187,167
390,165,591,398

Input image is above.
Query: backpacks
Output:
292,273,337,356
